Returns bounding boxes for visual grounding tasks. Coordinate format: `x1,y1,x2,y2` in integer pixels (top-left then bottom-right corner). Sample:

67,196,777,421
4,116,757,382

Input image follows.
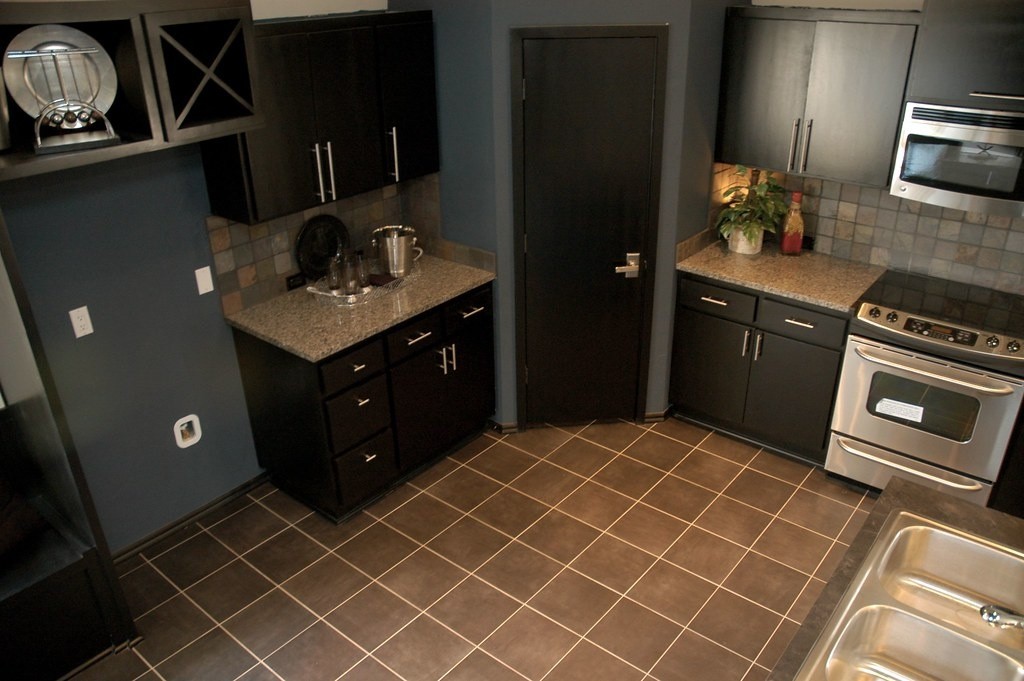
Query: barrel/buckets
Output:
370,225,423,278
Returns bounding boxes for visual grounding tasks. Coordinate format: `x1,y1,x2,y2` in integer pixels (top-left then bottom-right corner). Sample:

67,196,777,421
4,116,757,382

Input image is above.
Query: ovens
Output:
824,335,1024,508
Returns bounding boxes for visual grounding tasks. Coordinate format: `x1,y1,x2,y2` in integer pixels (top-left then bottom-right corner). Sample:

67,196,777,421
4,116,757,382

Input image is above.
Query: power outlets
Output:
68,305,95,339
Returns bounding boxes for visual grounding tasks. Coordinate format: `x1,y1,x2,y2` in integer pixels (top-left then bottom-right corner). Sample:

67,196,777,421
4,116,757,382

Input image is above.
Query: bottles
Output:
356,251,370,288
780,191,805,255
327,258,342,290
342,254,358,295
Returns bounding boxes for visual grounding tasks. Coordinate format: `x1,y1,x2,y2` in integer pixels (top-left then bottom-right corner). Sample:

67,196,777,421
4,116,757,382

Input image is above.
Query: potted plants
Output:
714,167,787,254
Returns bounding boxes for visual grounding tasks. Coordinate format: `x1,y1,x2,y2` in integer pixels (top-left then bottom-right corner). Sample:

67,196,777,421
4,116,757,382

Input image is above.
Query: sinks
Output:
874,506,1024,654
792,604,1024,681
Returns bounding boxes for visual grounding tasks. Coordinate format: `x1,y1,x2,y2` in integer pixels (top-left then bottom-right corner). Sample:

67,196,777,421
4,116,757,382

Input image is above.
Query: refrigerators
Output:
0,143,275,567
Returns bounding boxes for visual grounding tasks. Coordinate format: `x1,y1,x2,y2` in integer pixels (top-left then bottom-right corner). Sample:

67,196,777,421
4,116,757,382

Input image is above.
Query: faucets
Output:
979,605,1024,631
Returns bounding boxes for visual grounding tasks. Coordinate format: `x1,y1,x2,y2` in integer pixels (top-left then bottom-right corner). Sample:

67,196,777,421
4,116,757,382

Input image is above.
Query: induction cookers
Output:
848,269,1024,380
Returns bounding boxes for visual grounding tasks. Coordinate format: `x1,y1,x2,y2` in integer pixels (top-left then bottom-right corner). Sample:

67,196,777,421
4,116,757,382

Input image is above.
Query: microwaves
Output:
889,102,1024,218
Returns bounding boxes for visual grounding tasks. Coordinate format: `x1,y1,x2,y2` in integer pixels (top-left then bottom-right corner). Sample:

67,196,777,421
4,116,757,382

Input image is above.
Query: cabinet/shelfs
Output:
716,8,922,190
232,282,497,525
0,0,442,226
668,272,850,468
